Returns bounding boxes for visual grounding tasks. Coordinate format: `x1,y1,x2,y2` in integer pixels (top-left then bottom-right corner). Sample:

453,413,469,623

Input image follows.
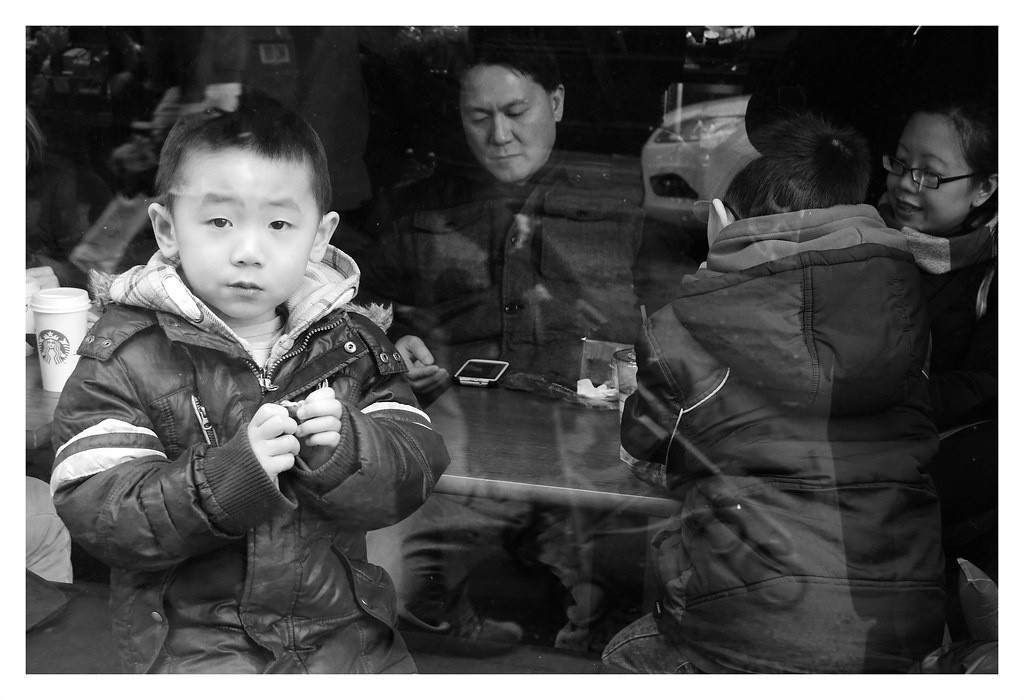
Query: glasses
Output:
693,197,742,224
883,155,977,190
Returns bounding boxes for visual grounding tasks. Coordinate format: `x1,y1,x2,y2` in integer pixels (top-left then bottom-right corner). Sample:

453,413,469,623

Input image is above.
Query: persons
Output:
49,102,450,674
357,38,646,650
605,107,998,673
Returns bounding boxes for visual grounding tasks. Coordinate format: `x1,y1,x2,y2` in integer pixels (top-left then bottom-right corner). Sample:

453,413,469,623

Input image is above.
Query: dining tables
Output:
26,353,63,451
423,383,683,617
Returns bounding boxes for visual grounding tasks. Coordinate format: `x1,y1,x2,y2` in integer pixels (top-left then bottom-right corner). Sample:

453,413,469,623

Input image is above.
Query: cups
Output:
579,339,636,388
611,348,642,468
702,31,719,45
29,287,92,392
26,266,61,358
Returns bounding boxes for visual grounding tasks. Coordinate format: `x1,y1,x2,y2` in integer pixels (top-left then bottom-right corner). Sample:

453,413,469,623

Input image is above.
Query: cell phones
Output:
453,359,510,388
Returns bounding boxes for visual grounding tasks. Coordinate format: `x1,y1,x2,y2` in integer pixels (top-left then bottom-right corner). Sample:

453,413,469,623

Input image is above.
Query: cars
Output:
637,91,766,239
